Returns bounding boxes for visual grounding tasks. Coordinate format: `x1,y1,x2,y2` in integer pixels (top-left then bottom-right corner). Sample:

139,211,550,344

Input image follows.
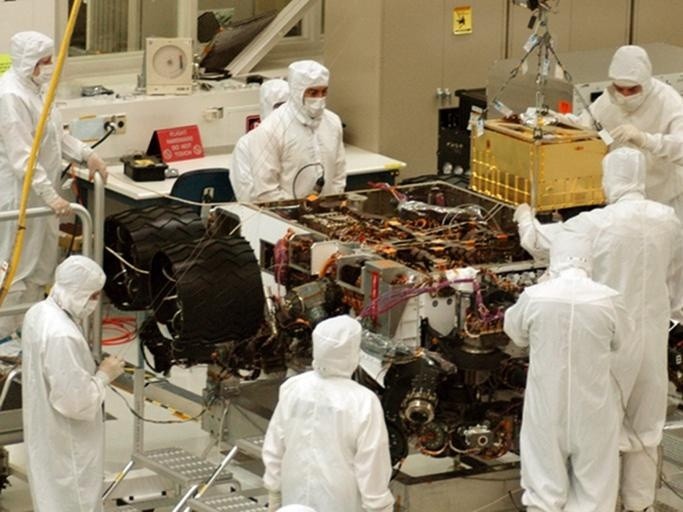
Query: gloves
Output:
513,204,535,222
609,124,645,149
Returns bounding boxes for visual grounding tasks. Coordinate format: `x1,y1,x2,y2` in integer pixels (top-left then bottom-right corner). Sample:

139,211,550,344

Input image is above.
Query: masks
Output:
32,64,54,84
80,300,97,318
304,97,326,118
614,91,643,112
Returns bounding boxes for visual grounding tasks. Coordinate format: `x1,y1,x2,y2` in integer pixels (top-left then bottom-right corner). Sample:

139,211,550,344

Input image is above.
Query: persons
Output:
503,228,637,512
511,146,682,512
252,58,347,203
530,43,682,207
263,314,394,512
19,255,125,512
228,77,291,207
0,31,109,345
